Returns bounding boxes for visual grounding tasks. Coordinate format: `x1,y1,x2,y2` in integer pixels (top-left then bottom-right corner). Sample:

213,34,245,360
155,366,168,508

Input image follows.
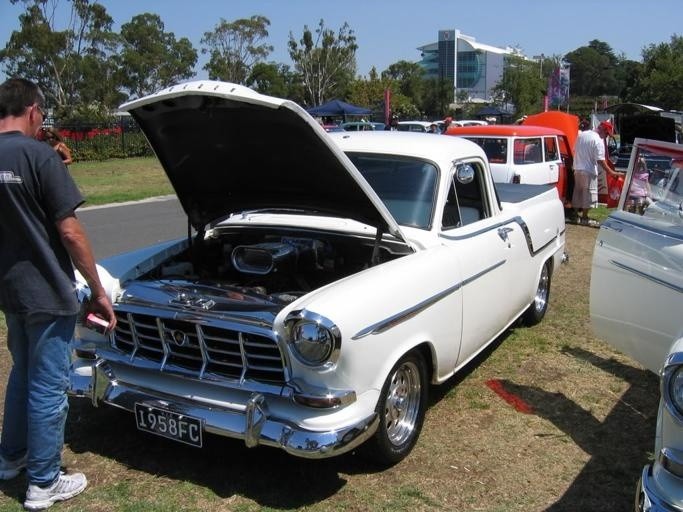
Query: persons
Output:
650,164,662,184
0,76,117,510
323,116,330,125
442,117,456,133
390,111,399,131
571,121,619,225
426,122,440,133
350,115,369,131
569,108,595,131
618,156,650,214
45,127,72,169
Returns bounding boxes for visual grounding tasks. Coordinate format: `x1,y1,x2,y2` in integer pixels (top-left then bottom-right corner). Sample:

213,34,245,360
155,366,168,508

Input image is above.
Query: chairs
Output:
24,470,87,510
0,450,29,480
570,215,598,226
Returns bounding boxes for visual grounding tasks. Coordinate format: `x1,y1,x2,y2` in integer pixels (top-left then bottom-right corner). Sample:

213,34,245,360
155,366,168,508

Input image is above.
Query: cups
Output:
27,103,48,121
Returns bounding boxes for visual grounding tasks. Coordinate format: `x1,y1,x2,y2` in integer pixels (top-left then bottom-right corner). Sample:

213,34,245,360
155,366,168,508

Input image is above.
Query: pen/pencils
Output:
603,104,681,172
442,111,579,210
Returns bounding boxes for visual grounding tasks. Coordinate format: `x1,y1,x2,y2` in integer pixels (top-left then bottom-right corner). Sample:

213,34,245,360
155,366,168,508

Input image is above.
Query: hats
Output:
434,119,489,126
392,121,441,135
68,79,566,466
587,136,681,509
325,122,391,131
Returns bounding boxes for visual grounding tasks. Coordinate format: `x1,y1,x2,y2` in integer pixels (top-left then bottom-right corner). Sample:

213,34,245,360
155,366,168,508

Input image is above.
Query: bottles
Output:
601,121,613,137
